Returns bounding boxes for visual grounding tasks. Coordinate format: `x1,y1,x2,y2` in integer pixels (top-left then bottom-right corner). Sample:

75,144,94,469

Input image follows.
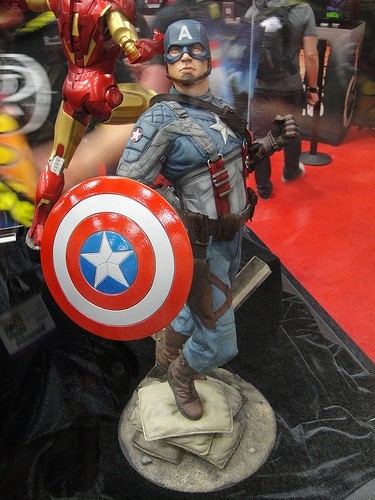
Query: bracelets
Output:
308,87,319,93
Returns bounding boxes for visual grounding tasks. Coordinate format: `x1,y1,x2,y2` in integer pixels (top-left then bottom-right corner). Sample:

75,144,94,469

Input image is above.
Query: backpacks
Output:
252,0,304,81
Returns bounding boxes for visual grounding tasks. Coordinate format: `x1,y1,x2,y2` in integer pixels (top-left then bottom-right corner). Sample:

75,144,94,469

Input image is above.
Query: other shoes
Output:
281,161,306,184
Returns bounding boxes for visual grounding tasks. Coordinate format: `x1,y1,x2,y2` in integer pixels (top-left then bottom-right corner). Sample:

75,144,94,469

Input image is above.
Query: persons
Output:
21,0,166,251
242,0,319,199
141,0,214,97
113,19,299,420
